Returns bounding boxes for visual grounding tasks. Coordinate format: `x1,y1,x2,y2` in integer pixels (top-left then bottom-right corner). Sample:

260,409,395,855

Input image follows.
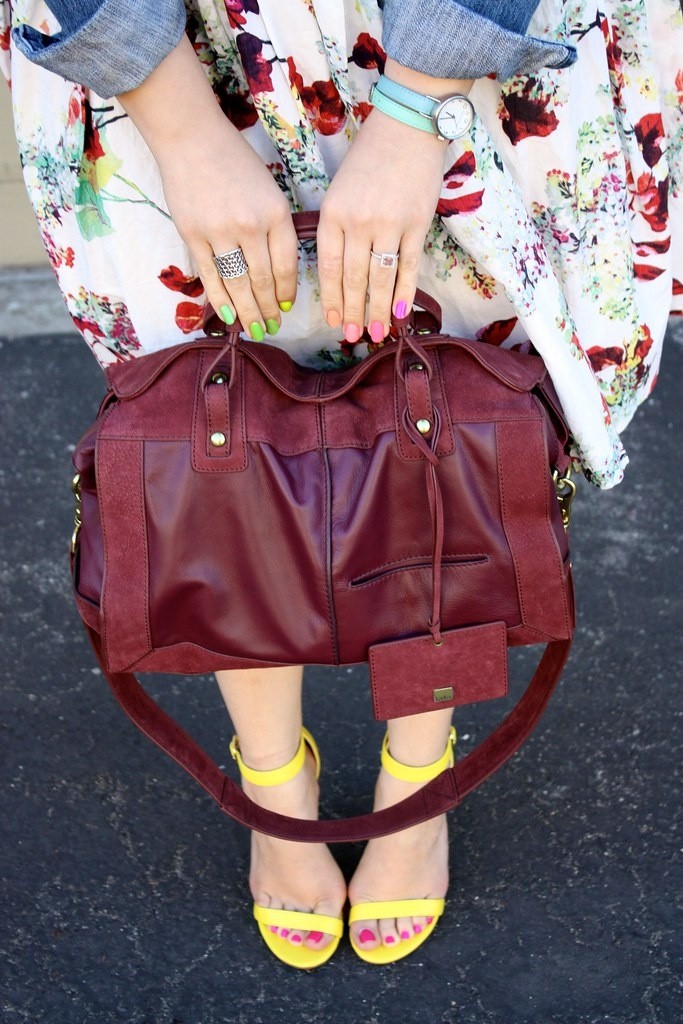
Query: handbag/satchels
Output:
68,209,579,844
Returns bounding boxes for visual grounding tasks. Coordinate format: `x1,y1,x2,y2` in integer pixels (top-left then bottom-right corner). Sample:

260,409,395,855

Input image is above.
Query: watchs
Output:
369,76,475,143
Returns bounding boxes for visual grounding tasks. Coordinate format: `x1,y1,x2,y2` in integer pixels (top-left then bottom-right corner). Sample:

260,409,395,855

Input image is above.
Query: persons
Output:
9,1,683,969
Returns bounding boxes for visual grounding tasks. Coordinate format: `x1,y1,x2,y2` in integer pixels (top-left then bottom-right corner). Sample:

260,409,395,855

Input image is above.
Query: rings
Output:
371,250,400,269
213,249,248,280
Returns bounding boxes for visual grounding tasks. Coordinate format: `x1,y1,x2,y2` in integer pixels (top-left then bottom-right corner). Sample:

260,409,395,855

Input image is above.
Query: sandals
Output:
347,725,455,965
229,725,347,969
